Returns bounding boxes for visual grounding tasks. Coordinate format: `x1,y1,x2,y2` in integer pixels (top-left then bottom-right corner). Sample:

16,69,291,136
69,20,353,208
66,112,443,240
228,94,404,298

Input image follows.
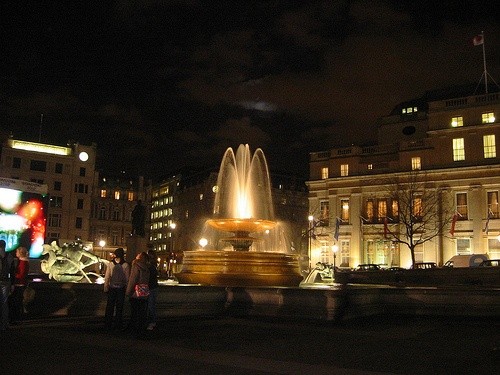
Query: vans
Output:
441,253,491,268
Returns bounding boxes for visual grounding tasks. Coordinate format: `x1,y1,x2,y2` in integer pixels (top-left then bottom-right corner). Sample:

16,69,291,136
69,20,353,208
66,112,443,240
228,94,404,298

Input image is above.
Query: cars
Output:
409,262,437,270
352,263,383,271
384,267,407,271
477,259,500,268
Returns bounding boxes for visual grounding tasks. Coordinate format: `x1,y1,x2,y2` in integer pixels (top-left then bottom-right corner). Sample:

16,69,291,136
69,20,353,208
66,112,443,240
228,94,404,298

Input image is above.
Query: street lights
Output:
170,223,177,277
332,245,338,274
100,240,105,277
308,215,313,272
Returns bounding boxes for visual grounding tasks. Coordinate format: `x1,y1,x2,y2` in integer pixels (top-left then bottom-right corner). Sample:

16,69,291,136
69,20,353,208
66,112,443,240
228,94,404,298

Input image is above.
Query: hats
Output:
0,240,5,248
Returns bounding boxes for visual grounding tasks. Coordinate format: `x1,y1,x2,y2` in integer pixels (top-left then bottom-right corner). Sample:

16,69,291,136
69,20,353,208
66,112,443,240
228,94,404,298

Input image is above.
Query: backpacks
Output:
108,259,128,288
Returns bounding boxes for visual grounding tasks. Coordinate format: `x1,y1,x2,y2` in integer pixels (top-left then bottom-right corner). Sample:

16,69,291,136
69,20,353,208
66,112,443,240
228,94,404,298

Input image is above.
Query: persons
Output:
104,248,130,330
132,200,147,235
0,240,30,330
120,250,157,332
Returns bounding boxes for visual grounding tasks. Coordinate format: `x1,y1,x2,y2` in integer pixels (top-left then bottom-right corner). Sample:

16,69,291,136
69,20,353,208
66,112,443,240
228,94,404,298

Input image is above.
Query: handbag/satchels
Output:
135,284,150,297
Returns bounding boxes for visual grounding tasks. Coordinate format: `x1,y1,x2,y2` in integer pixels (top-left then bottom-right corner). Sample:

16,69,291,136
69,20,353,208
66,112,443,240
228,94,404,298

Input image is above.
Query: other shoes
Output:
143,321,157,331
120,328,131,335
8,320,24,325
0,325,9,331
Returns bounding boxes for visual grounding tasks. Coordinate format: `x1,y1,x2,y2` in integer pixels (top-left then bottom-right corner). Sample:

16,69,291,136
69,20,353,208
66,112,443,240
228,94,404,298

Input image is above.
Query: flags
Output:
384,217,387,239
312,221,316,240
360,219,363,233
473,34,483,45
484,210,491,235
450,215,457,236
334,221,338,241
